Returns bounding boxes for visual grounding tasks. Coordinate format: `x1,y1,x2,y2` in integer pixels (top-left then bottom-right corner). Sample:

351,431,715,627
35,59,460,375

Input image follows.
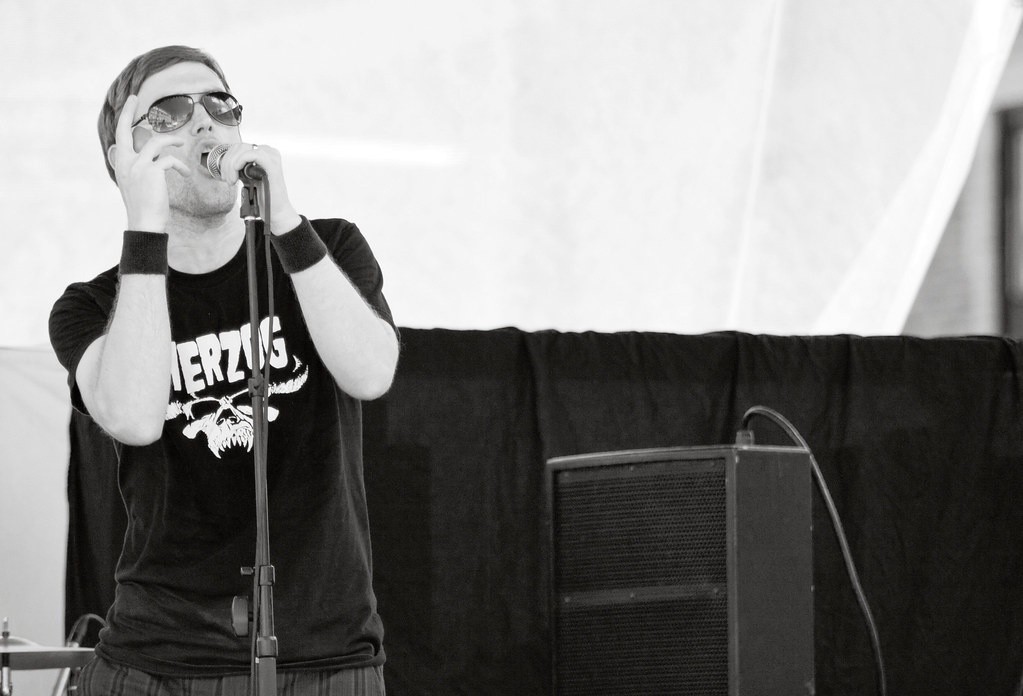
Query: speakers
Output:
545,444,817,695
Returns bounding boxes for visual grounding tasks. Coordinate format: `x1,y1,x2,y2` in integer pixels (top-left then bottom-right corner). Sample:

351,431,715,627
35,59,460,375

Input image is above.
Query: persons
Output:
48,45,400,696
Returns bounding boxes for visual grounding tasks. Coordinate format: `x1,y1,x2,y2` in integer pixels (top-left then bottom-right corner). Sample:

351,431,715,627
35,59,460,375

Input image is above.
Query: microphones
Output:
206,143,267,183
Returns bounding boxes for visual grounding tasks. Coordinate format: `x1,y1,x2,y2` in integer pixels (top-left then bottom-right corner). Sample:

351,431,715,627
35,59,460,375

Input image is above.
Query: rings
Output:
252,144,257,149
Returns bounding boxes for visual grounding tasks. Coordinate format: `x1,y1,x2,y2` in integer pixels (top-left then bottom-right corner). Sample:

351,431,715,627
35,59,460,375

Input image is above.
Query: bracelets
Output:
120,231,169,275
271,214,327,274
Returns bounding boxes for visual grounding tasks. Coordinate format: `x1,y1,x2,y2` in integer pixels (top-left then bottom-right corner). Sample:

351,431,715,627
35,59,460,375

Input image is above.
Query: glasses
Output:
130,91,243,135
